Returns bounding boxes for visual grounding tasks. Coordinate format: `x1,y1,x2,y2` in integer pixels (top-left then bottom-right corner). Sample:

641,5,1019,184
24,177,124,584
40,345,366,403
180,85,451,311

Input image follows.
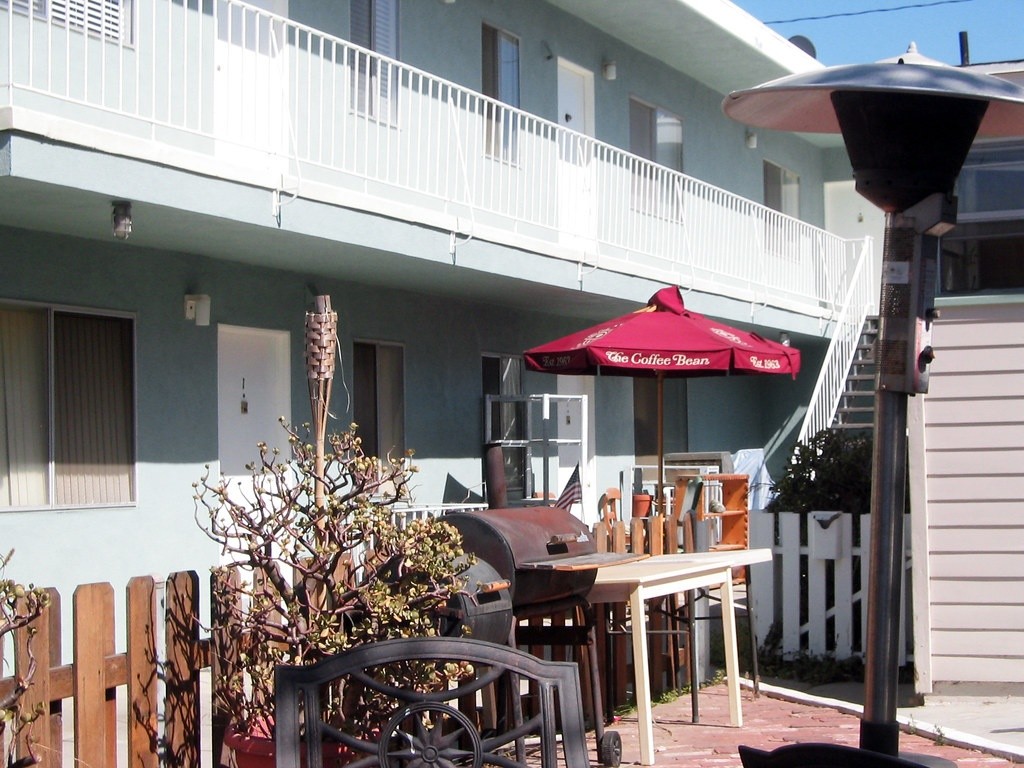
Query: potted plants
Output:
184,419,476,768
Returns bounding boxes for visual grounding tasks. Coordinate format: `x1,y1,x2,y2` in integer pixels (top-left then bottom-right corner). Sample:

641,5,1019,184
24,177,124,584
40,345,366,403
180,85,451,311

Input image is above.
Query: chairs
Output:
273,635,591,768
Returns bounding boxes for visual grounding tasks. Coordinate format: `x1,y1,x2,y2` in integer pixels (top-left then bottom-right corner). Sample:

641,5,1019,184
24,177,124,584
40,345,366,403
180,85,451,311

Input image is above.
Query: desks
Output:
585,548,773,767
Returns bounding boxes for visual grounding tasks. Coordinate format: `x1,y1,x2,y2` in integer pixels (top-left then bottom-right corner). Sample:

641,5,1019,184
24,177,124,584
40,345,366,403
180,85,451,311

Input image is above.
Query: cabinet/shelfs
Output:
673,471,761,722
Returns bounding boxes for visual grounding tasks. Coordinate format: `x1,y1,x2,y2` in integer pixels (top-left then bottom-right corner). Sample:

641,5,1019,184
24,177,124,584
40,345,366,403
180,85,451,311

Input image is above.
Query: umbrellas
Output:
523,289,800,518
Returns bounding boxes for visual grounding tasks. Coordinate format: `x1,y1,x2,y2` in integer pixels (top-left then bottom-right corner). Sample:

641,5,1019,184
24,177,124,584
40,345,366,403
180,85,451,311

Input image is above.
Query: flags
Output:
552,464,582,513
